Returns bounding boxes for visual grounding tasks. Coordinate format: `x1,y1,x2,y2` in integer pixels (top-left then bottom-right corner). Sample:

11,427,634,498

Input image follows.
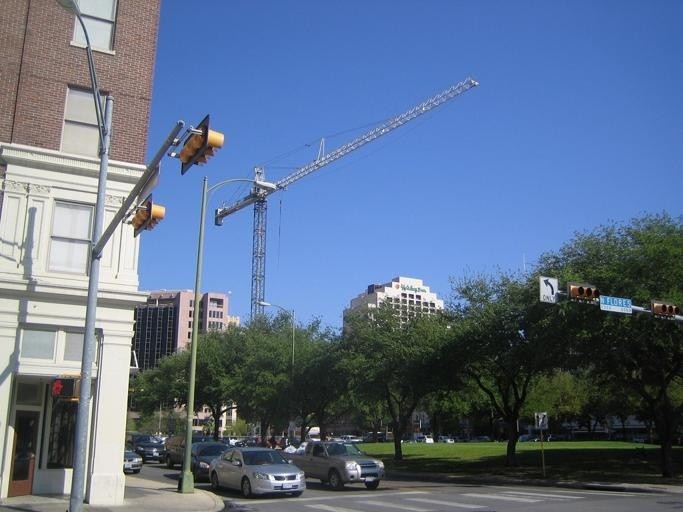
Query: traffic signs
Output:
539,276,559,304
600,296,633,314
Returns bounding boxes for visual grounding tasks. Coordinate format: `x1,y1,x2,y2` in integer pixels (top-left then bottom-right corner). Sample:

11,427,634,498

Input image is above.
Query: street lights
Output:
177,178,276,493
257,299,295,432
57,0,115,511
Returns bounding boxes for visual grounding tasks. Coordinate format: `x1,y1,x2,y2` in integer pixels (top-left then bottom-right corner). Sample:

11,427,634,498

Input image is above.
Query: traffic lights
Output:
132,204,166,232
650,299,679,322
567,282,600,306
180,130,224,166
51,378,77,399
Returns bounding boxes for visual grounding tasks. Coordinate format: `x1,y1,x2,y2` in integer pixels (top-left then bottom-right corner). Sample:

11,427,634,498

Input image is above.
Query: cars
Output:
123,433,165,473
164,435,306,499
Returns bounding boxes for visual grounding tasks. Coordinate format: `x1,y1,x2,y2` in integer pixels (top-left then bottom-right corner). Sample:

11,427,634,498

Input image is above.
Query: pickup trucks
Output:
276,440,384,490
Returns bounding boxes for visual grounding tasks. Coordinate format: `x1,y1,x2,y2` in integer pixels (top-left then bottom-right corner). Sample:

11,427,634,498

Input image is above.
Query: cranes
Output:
215,76,479,319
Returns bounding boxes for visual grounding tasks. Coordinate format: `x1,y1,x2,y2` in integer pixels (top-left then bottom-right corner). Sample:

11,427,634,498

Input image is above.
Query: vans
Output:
417,435,455,444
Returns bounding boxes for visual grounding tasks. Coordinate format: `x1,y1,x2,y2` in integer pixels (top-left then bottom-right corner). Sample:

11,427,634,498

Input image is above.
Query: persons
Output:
463,430,471,442
269,434,276,449
280,432,290,452
